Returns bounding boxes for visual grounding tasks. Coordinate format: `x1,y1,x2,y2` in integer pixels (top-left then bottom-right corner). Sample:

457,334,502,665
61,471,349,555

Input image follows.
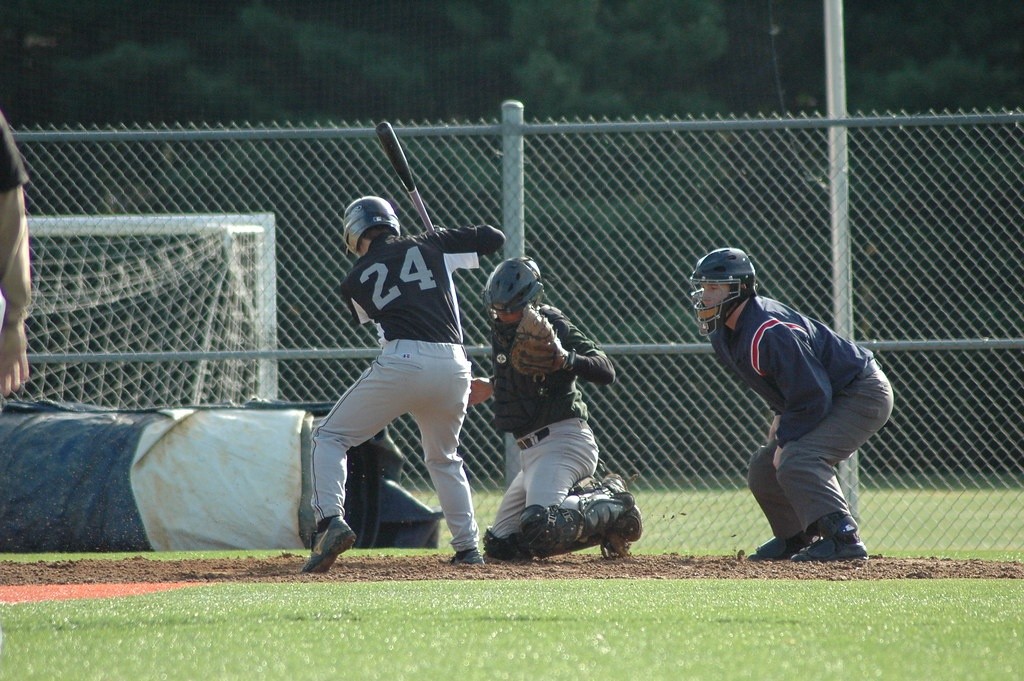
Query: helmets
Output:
342,195,400,255
480,258,545,336
689,247,755,335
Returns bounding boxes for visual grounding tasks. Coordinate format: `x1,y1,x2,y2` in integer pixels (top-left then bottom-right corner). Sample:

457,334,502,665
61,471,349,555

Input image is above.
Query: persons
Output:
693,247,894,560
468,256,642,557
304,196,507,574
0,113,32,394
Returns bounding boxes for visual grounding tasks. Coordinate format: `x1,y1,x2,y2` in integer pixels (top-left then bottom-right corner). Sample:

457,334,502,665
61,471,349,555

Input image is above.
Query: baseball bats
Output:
377,121,436,233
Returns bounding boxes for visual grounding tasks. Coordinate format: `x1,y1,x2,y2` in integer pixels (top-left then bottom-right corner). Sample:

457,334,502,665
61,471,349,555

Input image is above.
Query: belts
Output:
518,428,550,449
854,360,880,382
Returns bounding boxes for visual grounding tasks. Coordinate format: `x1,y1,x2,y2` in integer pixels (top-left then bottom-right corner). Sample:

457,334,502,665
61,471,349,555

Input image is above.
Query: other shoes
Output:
604,473,642,541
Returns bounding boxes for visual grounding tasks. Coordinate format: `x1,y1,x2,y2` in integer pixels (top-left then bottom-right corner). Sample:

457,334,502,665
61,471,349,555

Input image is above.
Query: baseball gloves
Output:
506,302,569,376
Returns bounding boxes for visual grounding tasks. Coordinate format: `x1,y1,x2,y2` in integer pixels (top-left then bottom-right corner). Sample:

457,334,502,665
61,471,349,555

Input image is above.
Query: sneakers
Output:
301,516,356,573
747,538,802,560
449,548,484,566
791,536,868,562
